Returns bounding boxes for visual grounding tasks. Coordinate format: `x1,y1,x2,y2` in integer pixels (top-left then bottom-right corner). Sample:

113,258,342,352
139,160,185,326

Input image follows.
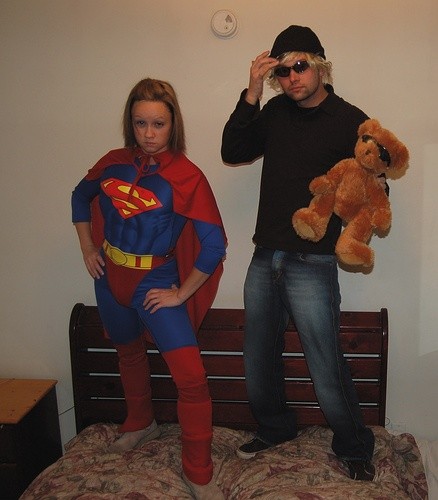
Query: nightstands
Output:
1,376,64,500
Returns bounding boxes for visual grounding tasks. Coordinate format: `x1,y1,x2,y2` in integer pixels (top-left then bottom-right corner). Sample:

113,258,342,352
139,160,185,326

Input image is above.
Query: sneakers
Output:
236,435,293,460
338,459,375,481
106,419,160,452
179,471,226,500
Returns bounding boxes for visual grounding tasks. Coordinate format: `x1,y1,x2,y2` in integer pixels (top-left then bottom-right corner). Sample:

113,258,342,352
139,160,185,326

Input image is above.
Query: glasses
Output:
272,61,309,76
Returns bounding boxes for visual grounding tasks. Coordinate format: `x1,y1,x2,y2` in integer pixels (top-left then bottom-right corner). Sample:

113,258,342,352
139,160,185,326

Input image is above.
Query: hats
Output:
269,25,326,60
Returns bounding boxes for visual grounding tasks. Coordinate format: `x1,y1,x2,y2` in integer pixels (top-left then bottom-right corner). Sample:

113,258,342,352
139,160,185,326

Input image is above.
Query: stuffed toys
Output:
291,118,409,268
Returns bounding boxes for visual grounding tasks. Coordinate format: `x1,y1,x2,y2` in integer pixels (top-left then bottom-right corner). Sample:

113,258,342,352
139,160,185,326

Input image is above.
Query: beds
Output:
22,303,431,500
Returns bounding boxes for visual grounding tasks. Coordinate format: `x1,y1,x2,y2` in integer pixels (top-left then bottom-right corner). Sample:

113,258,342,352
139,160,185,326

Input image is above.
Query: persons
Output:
220,23,390,479
71,78,228,500
